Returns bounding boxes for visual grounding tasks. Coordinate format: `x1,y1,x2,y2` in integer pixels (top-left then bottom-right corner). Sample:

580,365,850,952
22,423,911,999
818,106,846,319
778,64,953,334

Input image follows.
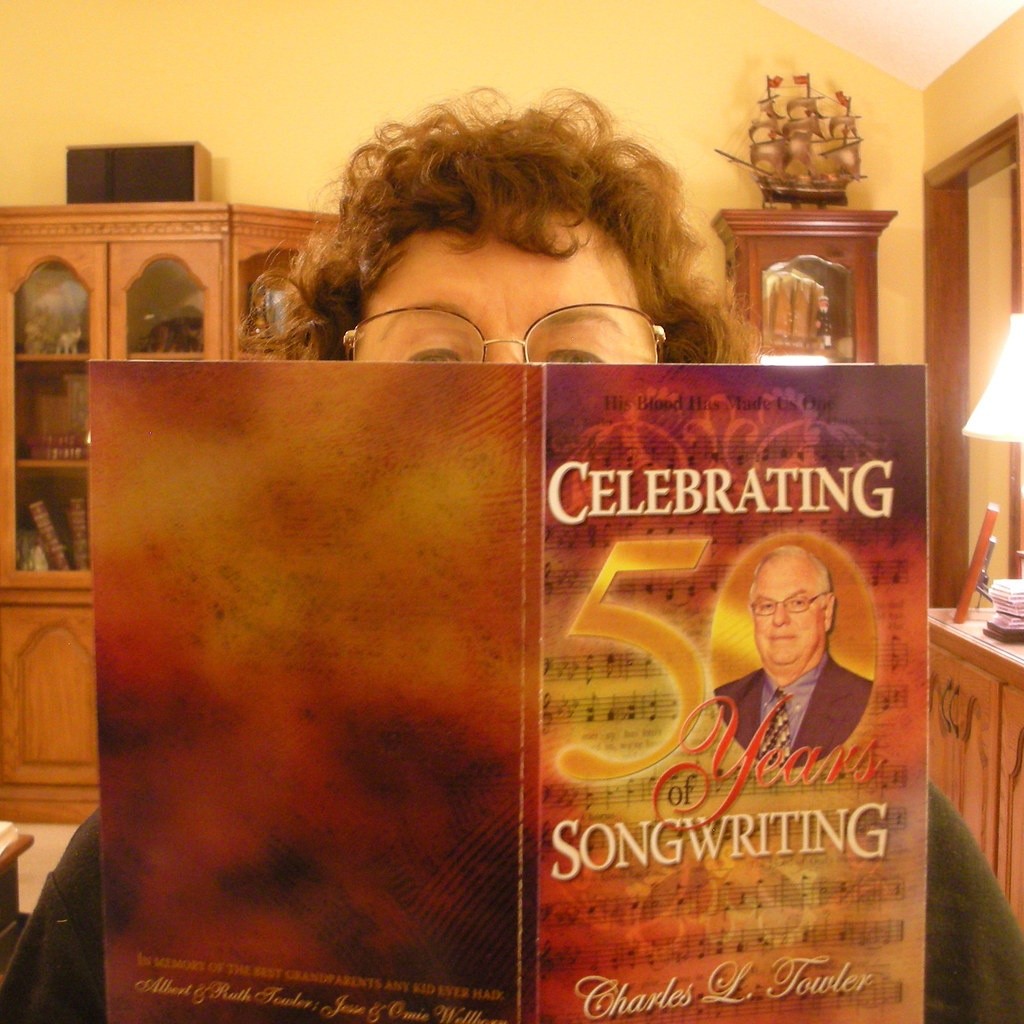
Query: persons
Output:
716,546,874,763
0,88,1024,1024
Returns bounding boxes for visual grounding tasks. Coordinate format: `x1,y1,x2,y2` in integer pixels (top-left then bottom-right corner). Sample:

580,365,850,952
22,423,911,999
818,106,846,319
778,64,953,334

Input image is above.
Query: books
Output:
87,364,925,1024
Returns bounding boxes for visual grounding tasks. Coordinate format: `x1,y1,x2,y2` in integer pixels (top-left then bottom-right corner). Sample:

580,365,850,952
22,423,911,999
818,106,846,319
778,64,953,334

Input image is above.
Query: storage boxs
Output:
66,142,211,203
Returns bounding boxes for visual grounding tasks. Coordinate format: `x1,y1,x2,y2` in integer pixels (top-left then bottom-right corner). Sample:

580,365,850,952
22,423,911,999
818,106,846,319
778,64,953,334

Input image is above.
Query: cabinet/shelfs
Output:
0,205,336,820
713,207,897,364
928,607,1024,930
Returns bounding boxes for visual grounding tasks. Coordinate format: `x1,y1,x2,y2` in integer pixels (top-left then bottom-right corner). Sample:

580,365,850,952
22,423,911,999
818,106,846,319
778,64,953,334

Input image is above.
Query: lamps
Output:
963,312,1024,643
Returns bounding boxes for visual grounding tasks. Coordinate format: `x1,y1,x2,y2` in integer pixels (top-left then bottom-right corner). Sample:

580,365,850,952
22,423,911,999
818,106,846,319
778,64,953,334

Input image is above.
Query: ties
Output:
762,693,792,766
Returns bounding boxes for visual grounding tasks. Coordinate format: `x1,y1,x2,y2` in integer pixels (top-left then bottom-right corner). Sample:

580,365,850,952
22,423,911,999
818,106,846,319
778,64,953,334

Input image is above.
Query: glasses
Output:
751,591,829,617
344,302,666,365
